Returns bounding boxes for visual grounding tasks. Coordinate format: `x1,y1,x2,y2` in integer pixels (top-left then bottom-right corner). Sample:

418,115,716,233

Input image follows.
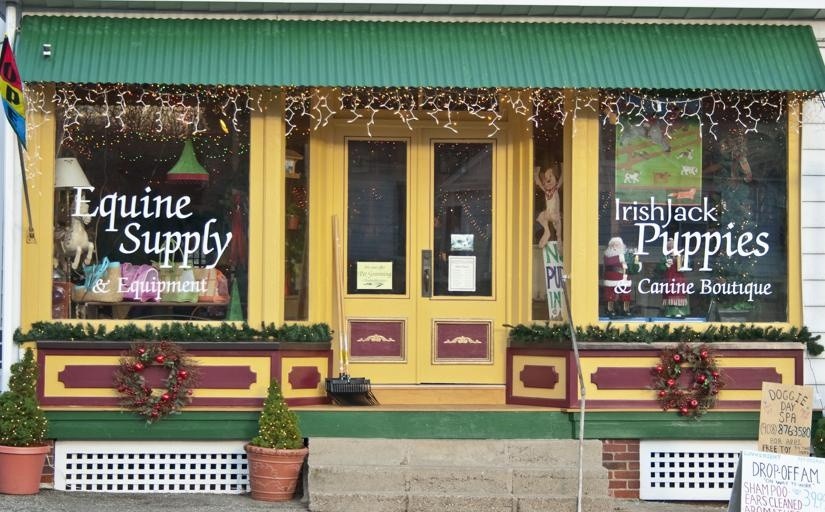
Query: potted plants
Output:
245,373,308,503
0,347,51,496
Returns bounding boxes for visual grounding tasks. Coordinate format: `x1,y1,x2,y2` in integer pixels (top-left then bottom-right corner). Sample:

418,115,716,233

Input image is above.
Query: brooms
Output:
324,213,380,406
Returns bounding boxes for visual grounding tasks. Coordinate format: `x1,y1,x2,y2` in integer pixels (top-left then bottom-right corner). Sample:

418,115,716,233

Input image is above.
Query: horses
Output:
56,191,95,272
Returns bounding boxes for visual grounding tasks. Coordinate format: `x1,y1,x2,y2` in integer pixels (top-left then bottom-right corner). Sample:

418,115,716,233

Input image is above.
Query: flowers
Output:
649,344,721,419
114,339,199,425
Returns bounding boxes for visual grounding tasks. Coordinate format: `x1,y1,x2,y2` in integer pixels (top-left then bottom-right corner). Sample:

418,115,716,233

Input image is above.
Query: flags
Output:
0,34,27,152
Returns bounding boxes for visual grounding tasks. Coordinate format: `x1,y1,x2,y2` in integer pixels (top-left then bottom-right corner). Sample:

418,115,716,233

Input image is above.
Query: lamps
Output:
165,116,213,183
56,157,93,316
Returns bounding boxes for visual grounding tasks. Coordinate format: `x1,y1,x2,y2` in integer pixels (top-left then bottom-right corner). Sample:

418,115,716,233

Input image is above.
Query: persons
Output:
601,236,631,316
534,162,563,253
664,240,691,317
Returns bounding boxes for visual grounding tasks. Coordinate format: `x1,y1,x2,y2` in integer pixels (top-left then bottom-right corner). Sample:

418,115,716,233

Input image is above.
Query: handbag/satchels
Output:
72,256,123,302
120,262,160,303
193,268,230,303
150,238,199,303
